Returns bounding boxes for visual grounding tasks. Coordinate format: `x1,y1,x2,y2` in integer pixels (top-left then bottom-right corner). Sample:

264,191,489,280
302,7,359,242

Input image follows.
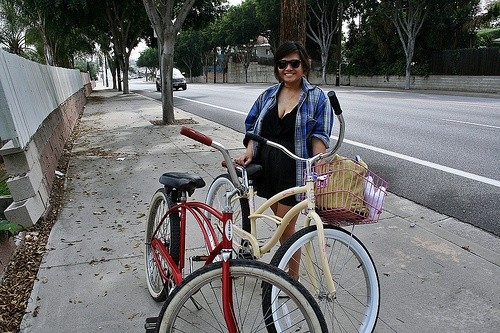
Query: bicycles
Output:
143,125,329,333
202,90,390,331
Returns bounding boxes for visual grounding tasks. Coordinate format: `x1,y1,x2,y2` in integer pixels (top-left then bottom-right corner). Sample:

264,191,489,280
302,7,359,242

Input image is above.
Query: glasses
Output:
277,59,302,69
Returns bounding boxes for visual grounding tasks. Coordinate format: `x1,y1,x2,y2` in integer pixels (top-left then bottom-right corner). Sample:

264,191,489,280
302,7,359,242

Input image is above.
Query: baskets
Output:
300,158,390,227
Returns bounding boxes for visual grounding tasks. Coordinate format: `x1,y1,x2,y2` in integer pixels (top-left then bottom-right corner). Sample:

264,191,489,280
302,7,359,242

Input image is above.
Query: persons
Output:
235,41,334,297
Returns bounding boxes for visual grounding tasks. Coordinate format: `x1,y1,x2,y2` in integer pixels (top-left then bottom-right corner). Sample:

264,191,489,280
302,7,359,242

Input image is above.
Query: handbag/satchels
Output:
309,153,386,224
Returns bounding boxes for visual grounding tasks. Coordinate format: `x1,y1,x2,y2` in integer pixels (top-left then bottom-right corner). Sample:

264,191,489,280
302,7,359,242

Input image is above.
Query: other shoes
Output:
277,290,289,298
285,268,289,272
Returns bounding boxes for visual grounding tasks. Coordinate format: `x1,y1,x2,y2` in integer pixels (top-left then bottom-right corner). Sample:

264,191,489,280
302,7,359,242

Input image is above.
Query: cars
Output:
156,68,187,91
